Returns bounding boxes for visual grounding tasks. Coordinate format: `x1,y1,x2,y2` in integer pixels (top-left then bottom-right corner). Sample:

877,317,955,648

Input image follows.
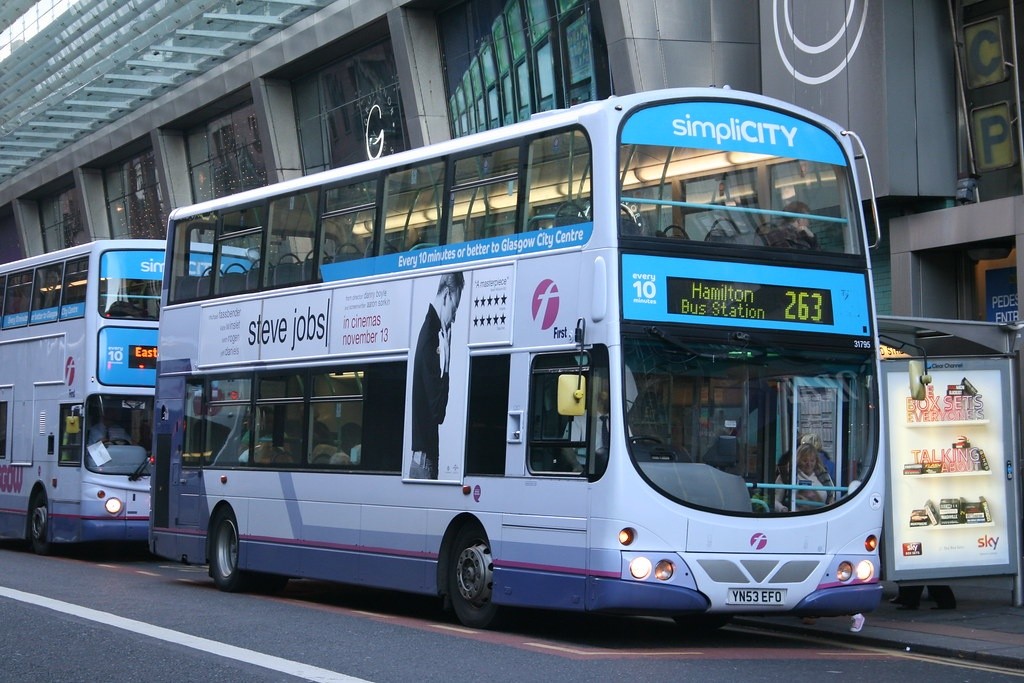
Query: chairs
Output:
273,452,331,464
174,203,791,301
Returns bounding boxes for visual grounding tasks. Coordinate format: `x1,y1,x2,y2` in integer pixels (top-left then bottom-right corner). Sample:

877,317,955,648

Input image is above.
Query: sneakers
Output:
849,613,865,632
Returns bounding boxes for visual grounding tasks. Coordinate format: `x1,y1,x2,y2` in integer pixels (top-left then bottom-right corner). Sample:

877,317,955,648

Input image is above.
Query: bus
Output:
1,239,263,555
149,81,889,632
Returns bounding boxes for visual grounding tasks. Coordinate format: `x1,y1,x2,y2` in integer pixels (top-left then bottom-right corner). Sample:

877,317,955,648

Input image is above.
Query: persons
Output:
409,271,465,479
775,444,835,513
850,614,865,632
88,403,133,446
888,586,956,610
801,433,835,487
768,201,820,251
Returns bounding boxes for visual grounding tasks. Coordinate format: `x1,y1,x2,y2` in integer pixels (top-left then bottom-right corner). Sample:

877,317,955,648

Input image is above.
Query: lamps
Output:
955,171,982,203
351,151,781,236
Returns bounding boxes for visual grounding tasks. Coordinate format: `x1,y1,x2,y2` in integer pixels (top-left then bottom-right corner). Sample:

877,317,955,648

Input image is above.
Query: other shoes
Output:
801,615,817,625
923,592,956,610
888,594,920,611
